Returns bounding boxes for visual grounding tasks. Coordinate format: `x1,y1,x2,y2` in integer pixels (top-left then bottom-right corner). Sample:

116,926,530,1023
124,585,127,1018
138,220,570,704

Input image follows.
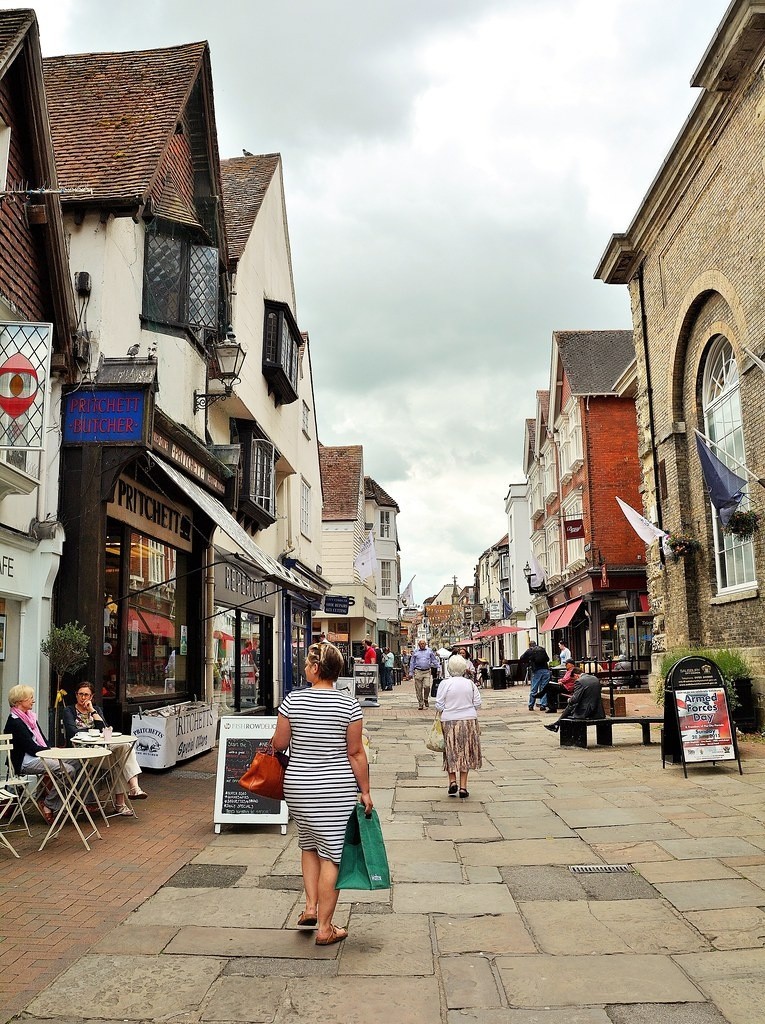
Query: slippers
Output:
316,924,350,945
297,909,318,927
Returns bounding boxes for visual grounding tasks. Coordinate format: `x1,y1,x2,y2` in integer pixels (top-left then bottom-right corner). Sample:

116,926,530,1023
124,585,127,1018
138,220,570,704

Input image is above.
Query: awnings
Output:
539,599,586,634
128,609,175,637
105,450,323,621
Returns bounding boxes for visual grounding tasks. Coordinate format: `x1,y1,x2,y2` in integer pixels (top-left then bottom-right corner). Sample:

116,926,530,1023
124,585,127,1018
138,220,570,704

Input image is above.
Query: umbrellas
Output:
470,626,527,666
454,640,479,650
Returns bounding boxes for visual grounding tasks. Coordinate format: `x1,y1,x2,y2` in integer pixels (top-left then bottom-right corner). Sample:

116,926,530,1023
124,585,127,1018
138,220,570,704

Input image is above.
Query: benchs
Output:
560,716,665,750
589,668,648,689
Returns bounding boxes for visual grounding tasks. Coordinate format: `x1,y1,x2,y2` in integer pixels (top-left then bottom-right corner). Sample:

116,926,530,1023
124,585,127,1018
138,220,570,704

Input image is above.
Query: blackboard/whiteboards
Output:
213,716,289,824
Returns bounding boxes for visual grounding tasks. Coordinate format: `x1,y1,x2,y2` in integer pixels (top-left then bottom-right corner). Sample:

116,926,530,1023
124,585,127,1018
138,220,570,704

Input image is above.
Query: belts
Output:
414,667,430,671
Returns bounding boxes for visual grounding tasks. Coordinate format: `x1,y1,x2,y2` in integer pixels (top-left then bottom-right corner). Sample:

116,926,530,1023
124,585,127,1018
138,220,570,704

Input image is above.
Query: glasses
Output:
566,663,569,665
76,692,91,698
318,641,331,661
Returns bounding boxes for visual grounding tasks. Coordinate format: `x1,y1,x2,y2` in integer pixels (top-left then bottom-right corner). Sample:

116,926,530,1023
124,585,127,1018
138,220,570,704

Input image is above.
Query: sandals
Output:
39,800,55,826
76,804,99,812
114,802,134,816
127,786,148,800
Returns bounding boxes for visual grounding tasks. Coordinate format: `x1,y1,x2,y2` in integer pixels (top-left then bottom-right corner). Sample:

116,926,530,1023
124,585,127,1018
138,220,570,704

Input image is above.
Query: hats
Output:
563,658,575,665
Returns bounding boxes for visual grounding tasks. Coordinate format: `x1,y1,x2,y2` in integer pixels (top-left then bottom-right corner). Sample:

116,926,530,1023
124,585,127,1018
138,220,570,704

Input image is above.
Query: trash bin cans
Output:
492,669,506,690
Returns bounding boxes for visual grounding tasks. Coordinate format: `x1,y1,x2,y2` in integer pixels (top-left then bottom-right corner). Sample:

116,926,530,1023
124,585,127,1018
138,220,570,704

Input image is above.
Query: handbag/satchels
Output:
431,673,443,697
335,804,391,891
426,711,444,752
237,735,289,800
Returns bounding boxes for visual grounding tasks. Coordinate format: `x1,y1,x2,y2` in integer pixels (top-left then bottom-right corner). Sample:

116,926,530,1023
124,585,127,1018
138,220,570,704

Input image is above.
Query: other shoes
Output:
544,724,559,732
532,693,542,699
545,708,558,713
529,706,534,711
385,688,392,691
418,702,423,710
540,707,546,711
424,700,429,707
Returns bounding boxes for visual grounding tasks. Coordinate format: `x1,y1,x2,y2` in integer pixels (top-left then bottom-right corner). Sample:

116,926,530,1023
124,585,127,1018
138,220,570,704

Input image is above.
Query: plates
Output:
73,733,122,741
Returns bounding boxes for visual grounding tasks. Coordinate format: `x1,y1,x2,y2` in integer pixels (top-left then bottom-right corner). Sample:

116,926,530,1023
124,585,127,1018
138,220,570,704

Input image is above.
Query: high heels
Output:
448,780,458,794
459,787,469,799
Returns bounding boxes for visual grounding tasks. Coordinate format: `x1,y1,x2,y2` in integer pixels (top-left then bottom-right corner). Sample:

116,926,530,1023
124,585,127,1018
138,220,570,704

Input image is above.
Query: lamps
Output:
523,560,531,583
193,324,247,416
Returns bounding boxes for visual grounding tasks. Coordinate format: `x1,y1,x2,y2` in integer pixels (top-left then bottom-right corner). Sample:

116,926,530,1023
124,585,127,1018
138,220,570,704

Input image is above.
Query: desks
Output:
391,667,402,686
34,746,112,852
130,700,220,771
70,732,141,827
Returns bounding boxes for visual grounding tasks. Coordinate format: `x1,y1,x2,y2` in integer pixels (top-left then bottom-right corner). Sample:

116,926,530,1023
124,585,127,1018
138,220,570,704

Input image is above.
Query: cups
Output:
89,729,100,735
75,732,89,738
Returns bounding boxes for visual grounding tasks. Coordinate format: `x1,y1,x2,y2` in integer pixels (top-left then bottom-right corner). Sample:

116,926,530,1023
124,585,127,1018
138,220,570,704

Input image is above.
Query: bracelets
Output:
91,710,97,717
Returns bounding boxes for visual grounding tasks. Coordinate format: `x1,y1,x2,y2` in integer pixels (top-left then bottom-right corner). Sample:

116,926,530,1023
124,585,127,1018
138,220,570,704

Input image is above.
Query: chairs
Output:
0,736,34,837
0,788,22,859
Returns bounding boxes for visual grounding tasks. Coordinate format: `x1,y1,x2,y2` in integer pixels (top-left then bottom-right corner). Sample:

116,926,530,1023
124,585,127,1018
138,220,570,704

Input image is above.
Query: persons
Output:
241,637,258,703
436,654,481,797
477,657,510,689
409,640,439,710
63,682,148,814
273,640,374,946
431,646,476,682
4,685,98,823
521,641,632,732
361,639,409,691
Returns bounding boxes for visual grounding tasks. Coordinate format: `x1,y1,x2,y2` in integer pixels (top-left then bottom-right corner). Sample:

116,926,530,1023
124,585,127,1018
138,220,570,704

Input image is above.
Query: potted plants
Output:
655,641,758,734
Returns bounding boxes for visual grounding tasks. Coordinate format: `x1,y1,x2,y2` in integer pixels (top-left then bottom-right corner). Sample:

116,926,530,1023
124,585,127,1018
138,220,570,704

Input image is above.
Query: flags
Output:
402,581,414,610
528,554,547,594
616,497,672,555
355,526,378,582
503,599,512,619
696,435,746,527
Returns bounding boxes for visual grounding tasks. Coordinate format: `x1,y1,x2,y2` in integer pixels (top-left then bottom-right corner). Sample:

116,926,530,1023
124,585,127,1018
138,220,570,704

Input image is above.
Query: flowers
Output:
666,531,700,564
720,508,761,539
